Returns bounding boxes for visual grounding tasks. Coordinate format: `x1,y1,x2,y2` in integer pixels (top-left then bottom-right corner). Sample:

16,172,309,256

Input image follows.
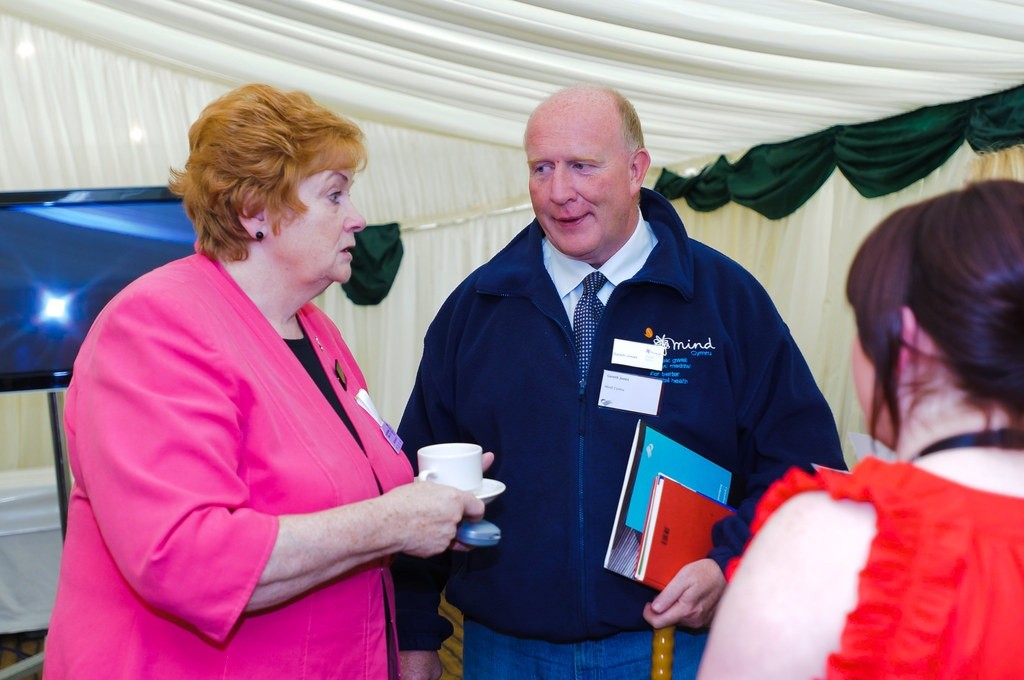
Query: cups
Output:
418,443,483,492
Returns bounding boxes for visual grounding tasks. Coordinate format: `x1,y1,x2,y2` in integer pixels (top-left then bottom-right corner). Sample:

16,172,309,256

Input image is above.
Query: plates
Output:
415,476,506,501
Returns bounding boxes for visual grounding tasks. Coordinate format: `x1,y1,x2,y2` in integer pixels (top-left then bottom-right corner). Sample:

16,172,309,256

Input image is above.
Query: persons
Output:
389,85,849,678
695,178,1024,680
33,82,495,680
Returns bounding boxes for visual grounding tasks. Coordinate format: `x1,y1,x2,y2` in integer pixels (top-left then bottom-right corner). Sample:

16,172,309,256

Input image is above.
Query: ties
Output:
572,272,609,388
